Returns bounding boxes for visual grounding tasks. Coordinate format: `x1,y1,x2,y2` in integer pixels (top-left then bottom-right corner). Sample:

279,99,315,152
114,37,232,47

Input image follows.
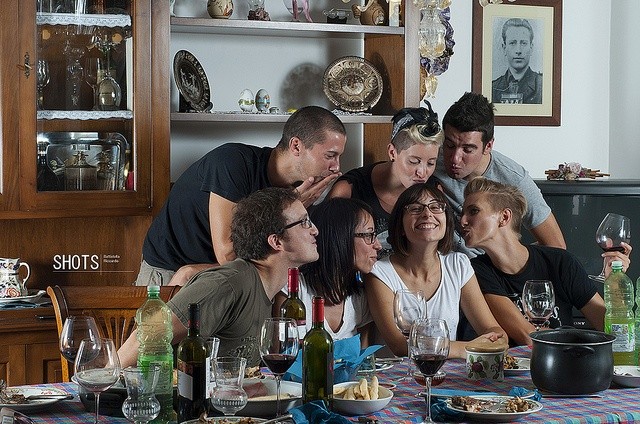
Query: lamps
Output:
411,1,447,98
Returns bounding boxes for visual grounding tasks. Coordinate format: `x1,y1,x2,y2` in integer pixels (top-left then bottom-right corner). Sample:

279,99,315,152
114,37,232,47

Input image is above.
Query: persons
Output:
460,177,634,346
273,198,382,352
133,106,348,286
492,17,543,105
433,92,568,258
365,182,508,359
100,185,319,371
324,100,444,261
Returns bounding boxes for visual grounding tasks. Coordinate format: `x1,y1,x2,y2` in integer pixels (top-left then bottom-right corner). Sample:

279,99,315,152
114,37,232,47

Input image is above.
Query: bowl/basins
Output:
464,342,506,382
210,377,302,419
71,368,171,418
331,381,393,416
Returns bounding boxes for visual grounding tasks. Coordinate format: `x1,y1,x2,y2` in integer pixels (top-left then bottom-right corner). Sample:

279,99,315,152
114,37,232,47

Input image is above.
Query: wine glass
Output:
121,366,161,424
413,363,446,391
411,318,451,424
258,318,300,424
36,59,51,111
203,336,220,384
522,280,555,331
59,316,102,398
74,338,121,424
393,289,428,384
588,212,630,285
210,356,248,416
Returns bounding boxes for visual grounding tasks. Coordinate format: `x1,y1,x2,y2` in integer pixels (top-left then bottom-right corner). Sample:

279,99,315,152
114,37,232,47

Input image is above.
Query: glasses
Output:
401,201,446,215
354,232,376,244
285,215,312,229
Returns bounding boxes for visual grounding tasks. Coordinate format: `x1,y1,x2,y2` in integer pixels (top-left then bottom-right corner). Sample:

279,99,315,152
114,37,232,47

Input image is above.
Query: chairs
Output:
354,318,375,355
45,282,182,383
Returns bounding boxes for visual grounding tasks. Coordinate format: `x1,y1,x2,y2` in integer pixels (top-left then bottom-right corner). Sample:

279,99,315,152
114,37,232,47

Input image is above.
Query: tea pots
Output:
0,255,31,298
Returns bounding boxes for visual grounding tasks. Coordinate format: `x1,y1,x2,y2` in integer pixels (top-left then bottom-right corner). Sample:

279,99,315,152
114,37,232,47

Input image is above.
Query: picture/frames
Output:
471,1,563,127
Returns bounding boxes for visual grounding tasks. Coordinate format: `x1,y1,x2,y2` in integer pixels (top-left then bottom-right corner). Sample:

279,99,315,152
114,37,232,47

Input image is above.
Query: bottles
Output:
96,55,121,110
635,276,640,376
278,268,307,354
177,303,209,423
301,297,335,413
603,261,634,367
135,284,174,423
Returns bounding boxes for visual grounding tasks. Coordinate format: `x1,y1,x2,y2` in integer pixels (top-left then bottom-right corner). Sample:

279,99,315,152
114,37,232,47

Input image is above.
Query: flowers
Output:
544,160,609,181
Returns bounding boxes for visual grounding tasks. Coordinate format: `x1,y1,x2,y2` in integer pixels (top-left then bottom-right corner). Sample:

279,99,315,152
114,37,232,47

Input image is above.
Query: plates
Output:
180,415,281,424
446,395,544,422
322,55,384,112
0,385,68,413
0,288,46,307
503,357,531,376
355,354,394,376
173,50,210,111
610,365,640,388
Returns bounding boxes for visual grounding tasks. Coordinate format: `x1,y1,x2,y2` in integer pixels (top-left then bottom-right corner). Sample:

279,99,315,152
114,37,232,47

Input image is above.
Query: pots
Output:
527,329,617,396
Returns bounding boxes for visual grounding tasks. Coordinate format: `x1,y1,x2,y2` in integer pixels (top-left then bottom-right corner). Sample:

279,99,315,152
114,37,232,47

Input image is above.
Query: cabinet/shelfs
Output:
27,329,138,386
152,0,421,209
1,0,171,222
0,330,26,388
